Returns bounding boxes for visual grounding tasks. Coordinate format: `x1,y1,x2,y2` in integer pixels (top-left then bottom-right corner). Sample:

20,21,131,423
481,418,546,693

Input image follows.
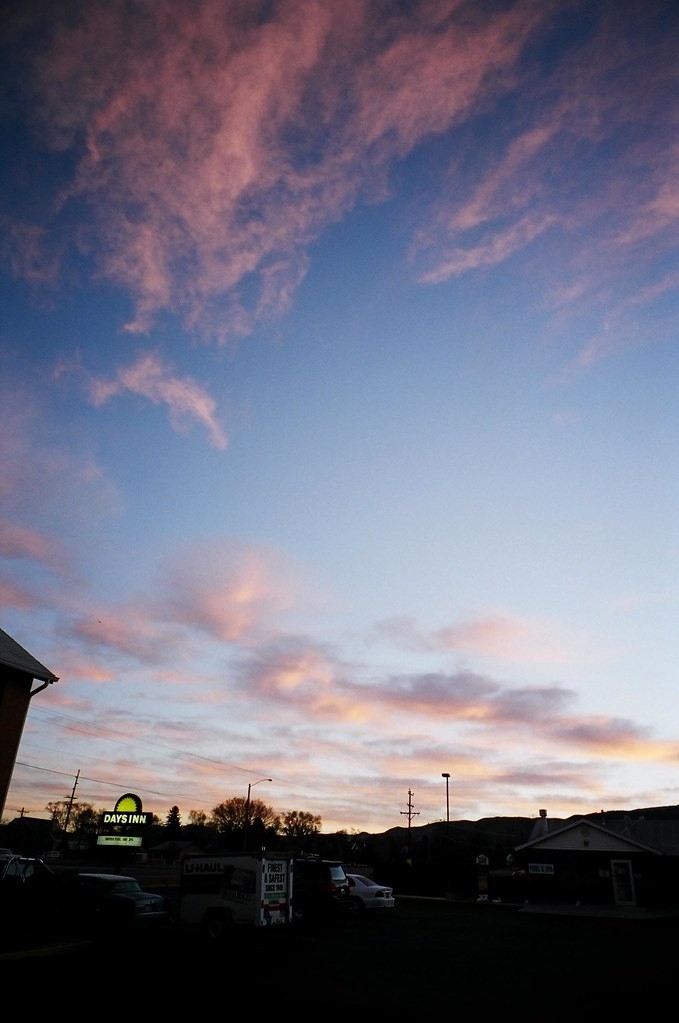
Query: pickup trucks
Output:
0,848,22,864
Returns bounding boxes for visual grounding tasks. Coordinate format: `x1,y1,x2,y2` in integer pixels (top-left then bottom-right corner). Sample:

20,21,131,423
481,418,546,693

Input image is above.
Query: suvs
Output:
68,873,169,927
293,857,351,923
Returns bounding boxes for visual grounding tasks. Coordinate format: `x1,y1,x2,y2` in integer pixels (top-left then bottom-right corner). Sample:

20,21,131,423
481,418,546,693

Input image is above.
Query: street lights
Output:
244,779,272,856
442,774,450,823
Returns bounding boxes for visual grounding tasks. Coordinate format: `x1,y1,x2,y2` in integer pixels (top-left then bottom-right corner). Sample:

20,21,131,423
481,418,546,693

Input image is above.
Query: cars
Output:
345,873,395,917
